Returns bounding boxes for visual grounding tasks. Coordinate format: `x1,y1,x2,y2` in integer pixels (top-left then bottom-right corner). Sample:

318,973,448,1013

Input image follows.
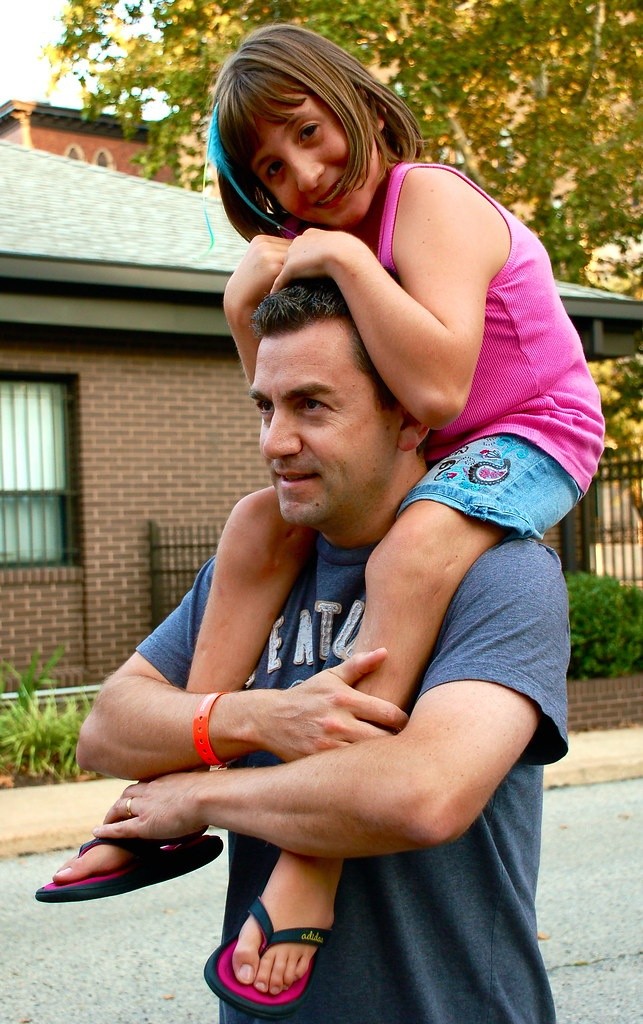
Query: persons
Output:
78,273,574,1023
35,21,606,1019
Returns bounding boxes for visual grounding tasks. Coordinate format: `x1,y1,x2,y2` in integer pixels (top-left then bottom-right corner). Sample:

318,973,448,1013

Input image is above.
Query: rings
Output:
124,795,134,817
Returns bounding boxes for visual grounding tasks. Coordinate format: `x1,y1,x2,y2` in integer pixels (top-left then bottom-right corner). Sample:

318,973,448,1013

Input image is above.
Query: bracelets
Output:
192,691,237,772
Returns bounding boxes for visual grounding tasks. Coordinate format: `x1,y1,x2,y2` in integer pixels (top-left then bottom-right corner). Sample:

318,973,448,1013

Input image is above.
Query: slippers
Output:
205,896,331,1019
35,829,223,903
